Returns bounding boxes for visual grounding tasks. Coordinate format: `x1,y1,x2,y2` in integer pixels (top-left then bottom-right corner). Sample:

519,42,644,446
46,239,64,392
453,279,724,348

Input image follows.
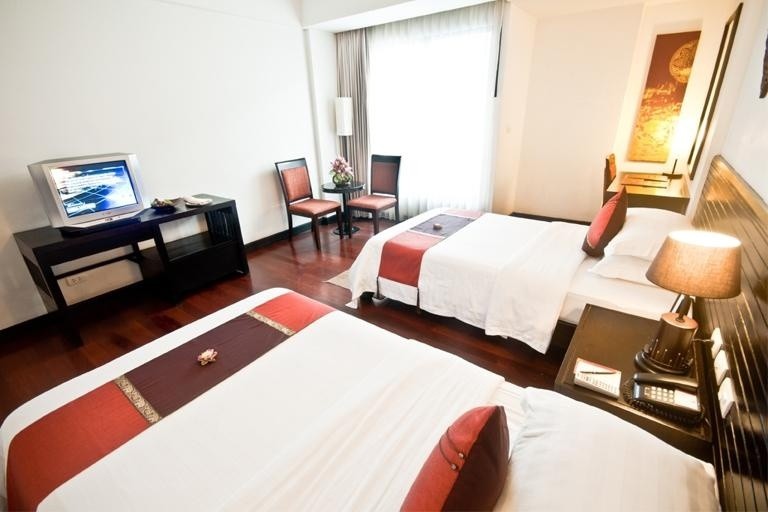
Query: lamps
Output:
335,97,353,161
663,138,691,179
635,231,741,373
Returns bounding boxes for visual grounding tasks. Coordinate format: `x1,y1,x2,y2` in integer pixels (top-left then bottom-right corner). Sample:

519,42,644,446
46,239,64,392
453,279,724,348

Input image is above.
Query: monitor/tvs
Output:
25,152,145,232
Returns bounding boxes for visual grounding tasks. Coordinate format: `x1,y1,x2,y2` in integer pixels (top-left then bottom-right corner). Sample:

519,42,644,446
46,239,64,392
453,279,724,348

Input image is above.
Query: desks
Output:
322,180,365,234
606,174,690,215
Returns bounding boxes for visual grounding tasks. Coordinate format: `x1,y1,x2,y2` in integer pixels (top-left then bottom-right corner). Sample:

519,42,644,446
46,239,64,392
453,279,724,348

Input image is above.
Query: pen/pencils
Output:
580,371,617,374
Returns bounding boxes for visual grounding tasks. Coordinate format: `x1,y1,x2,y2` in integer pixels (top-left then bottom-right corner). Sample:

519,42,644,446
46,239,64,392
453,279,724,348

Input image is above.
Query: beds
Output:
327,210,695,354
0,286,722,512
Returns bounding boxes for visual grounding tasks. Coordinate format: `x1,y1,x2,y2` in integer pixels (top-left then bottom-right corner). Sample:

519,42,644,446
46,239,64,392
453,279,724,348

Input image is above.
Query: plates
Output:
185,199,212,206
151,198,180,208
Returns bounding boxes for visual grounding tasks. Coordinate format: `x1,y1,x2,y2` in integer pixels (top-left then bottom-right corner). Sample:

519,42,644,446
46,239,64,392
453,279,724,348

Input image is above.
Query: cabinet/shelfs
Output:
12,194,249,348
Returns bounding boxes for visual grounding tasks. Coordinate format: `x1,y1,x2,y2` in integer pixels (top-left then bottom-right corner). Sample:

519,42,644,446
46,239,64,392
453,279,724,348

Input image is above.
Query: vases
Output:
333,173,350,187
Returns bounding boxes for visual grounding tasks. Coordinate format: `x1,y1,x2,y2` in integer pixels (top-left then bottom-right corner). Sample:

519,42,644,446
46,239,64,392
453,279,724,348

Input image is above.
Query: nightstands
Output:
554,304,713,461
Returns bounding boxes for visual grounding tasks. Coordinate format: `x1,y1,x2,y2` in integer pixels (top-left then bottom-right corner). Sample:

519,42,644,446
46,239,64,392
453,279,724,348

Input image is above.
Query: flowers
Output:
330,158,354,177
198,349,217,365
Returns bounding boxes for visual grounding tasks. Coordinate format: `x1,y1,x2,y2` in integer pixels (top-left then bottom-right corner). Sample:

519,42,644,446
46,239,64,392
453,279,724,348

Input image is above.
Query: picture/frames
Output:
686,2,743,179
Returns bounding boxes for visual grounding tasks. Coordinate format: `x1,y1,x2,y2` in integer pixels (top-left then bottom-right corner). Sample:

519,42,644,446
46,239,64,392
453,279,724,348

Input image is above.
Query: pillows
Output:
603,207,695,261
582,186,628,255
493,387,722,511
399,406,509,512
589,256,655,286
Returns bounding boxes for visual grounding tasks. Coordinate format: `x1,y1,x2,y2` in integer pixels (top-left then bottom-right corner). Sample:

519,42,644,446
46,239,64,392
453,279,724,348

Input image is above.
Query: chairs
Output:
346,154,401,236
275,158,343,251
602,155,617,206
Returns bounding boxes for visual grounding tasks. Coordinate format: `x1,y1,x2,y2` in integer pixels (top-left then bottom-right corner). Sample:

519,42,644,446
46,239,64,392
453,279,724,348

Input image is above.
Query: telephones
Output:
633,372,702,426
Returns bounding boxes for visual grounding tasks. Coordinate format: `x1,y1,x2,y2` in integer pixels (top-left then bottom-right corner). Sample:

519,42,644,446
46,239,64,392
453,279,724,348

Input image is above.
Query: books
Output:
571,356,622,399
